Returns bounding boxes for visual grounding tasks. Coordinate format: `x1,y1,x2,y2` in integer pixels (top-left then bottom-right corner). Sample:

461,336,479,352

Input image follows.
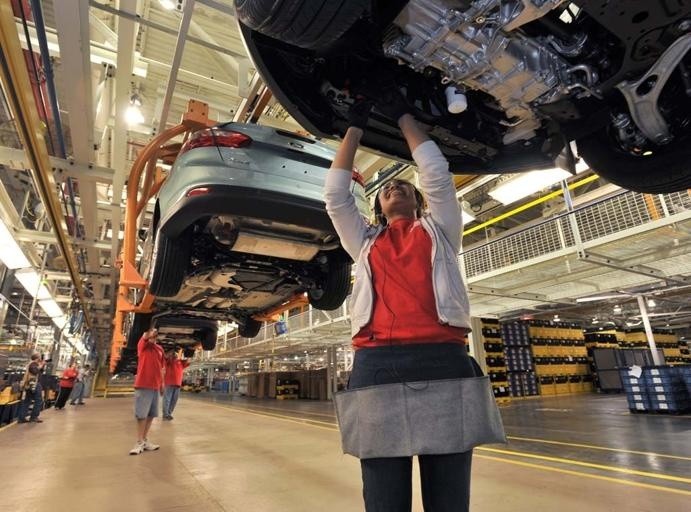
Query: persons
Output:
129,327,167,455
18,353,47,423
69,364,91,406
162,348,191,421
54,361,79,411
324,88,508,512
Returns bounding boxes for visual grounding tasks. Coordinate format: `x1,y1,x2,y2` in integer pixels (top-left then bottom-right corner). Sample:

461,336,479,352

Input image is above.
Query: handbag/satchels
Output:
24,376,38,391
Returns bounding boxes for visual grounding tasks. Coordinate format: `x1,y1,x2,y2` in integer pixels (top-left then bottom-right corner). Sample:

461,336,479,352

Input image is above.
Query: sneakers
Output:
143,438,159,450
129,442,143,454
55,406,65,410
30,417,43,422
18,418,29,423
164,415,174,420
70,402,84,405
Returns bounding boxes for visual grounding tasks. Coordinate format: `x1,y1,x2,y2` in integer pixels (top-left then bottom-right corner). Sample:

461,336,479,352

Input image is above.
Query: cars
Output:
234,0,690,194
